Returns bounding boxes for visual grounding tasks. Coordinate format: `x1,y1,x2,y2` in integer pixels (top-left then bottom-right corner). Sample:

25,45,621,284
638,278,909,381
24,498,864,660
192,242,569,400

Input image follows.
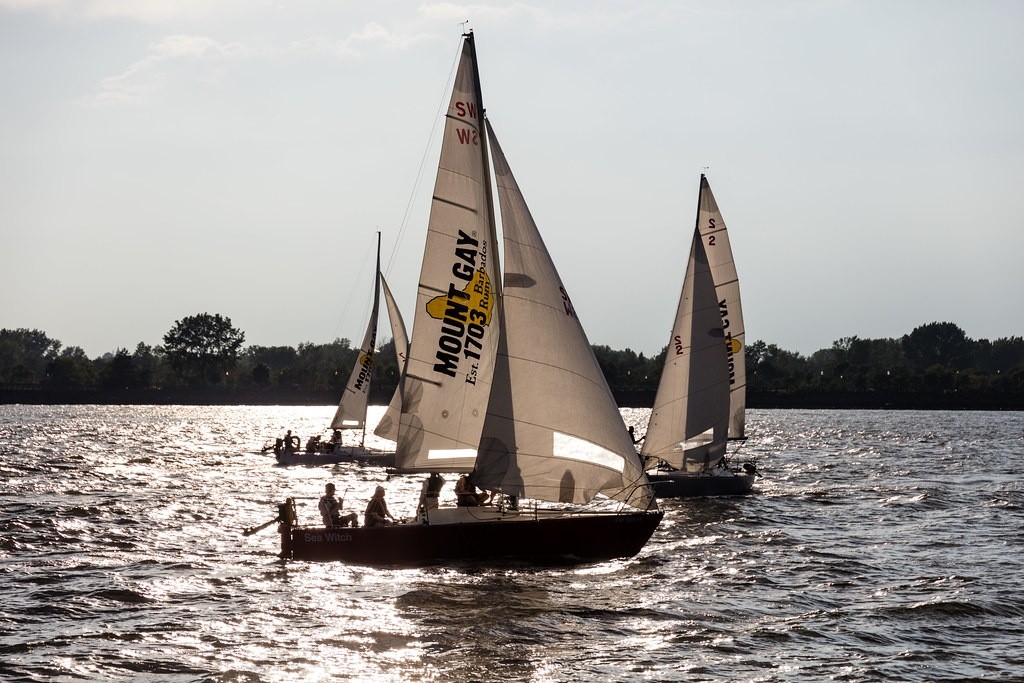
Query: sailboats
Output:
639,174,754,498
274,232,409,463
294,27,666,565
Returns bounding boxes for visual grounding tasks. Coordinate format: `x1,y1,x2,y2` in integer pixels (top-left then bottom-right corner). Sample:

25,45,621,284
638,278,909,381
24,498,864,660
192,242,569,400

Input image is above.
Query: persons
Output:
628,426,645,443
418,472,526,510
319,483,359,528
275,430,343,453
365,486,395,528
